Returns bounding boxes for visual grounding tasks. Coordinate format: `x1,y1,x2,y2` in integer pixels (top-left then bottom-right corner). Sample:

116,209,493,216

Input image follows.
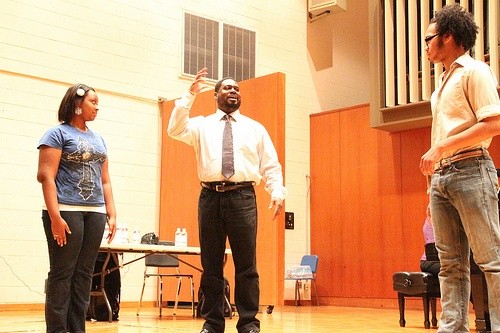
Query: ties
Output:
221,115,235,178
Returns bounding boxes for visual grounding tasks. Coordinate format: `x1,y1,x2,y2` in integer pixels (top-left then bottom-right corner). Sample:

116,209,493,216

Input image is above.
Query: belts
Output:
202,182,251,193
433,150,489,171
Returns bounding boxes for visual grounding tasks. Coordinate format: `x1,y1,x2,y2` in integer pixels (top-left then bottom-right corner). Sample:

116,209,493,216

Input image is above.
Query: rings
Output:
54,234,58,237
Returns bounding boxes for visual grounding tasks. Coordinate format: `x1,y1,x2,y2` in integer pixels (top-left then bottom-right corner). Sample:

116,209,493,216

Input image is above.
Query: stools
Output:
393,272,440,328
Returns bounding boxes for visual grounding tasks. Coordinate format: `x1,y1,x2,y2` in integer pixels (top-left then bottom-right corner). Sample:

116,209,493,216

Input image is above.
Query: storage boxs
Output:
285,266,314,279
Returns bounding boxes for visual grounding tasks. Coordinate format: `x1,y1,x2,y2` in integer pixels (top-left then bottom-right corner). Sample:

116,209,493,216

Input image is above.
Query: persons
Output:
417,3,500,333
168,67,287,333
420,178,474,310
37,84,116,333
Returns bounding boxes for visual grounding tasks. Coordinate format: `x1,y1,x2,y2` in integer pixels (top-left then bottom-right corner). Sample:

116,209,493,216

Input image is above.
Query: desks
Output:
90,243,232,320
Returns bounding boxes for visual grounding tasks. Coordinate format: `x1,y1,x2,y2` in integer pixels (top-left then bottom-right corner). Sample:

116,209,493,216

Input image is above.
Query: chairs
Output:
285,254,320,307
136,241,196,318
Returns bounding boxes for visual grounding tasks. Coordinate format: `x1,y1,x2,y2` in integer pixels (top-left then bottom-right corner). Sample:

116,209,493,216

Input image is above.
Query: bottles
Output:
175,228,181,247
101,224,129,243
286,266,312,278
134,227,140,244
181,228,187,247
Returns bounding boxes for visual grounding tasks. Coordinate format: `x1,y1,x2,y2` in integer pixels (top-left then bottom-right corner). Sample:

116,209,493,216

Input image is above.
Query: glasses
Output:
424,31,445,45
